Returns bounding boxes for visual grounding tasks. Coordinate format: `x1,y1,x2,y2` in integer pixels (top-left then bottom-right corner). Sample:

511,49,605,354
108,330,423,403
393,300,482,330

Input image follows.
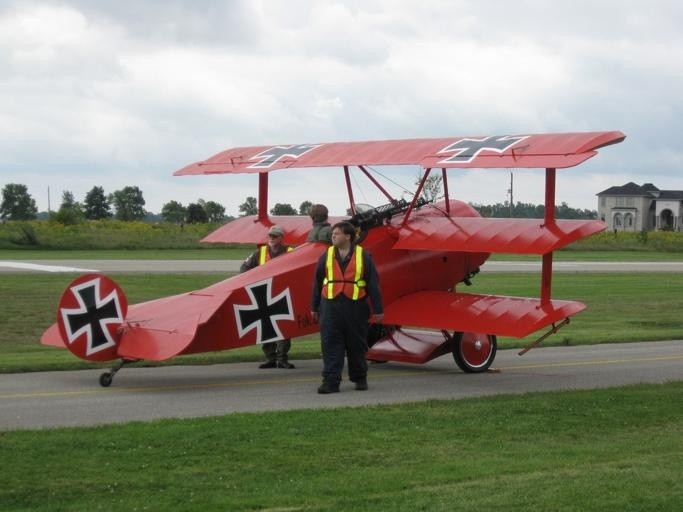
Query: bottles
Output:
267,225,284,237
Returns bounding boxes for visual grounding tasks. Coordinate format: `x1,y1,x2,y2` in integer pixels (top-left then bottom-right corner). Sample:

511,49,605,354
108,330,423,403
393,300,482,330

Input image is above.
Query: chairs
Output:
276,360,295,369
259,359,276,369
317,381,341,394
355,379,368,391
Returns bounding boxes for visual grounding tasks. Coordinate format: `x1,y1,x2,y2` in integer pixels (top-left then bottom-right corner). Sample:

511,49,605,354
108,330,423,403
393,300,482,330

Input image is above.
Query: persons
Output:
308,220,385,394
304,202,333,245
239,224,299,370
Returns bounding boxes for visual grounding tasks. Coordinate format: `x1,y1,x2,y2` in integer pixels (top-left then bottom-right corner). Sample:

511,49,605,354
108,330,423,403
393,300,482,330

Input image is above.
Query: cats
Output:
39,132,626,387
